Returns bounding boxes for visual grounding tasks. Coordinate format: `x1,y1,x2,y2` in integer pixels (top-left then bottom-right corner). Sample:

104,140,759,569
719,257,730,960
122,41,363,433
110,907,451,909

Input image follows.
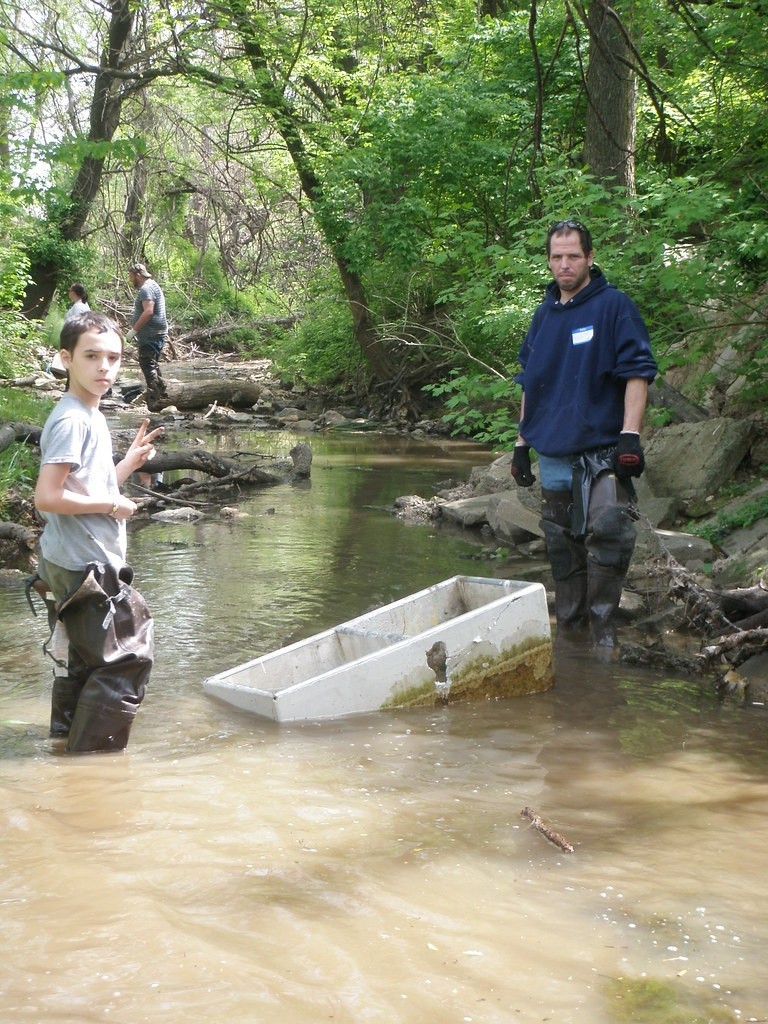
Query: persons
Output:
126,264,168,387
510,220,659,645
62,284,112,395
25,312,165,752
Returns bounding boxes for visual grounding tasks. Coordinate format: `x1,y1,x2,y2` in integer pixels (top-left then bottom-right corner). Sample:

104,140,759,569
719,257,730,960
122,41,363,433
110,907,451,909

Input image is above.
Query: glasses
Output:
546,220,588,235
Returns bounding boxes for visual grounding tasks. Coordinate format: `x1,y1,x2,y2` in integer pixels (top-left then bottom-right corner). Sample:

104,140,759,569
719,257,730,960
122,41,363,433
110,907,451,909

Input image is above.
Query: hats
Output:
128,263,153,278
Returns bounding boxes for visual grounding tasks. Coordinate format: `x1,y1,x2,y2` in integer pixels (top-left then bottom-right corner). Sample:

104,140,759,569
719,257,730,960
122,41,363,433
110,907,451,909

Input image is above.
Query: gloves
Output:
511,445,536,487
125,328,138,343
610,430,646,477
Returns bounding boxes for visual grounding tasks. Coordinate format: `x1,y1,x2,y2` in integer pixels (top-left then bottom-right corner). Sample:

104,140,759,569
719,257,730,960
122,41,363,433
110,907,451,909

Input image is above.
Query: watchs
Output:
108,501,118,516
